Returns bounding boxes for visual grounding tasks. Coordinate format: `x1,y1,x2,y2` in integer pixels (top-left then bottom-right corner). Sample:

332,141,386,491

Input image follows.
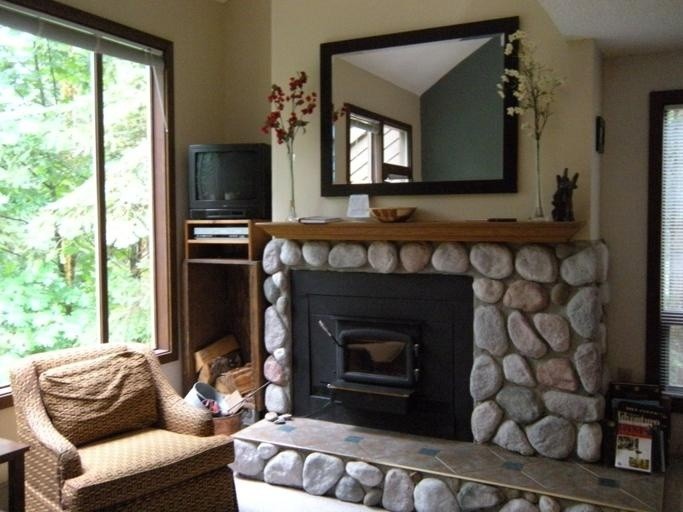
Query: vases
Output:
527,140,554,223
285,153,300,222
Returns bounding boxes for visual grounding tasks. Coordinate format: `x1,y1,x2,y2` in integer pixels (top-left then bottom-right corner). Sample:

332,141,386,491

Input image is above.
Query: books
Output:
602,381,668,472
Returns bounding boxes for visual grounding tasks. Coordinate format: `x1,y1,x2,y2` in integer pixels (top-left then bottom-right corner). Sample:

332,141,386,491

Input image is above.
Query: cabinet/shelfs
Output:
181,218,272,427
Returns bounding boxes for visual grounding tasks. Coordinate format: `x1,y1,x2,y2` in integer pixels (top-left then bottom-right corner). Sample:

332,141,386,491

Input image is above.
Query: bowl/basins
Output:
213,410,244,436
368,206,417,223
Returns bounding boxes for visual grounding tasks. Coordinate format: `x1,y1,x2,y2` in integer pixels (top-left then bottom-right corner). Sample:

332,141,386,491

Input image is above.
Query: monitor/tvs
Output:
188,142,271,219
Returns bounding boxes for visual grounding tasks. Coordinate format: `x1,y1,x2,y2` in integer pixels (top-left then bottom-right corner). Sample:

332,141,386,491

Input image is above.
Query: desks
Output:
0,436,31,512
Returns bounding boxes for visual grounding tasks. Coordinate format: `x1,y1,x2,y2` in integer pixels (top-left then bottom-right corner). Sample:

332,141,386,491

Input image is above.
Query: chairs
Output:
9,342,240,512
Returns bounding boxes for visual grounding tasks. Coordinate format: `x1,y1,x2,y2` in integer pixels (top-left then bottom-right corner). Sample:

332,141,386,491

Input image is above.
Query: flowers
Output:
261,71,318,207
496,29,565,219
330,102,351,121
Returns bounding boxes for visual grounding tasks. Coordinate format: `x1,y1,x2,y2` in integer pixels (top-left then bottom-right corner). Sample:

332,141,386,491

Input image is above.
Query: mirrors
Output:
320,16,520,196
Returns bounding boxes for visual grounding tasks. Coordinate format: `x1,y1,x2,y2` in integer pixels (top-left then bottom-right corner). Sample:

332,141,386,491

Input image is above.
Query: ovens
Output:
291,266,474,444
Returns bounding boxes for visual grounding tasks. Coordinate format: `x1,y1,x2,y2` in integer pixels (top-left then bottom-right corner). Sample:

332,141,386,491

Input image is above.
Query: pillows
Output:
37,352,159,446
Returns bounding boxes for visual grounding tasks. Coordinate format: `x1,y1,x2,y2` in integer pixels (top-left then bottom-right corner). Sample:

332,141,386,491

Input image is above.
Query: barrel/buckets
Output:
183,382,224,416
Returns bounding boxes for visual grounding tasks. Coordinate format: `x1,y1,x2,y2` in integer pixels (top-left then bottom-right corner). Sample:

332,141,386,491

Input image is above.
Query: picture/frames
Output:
596,116,605,153
